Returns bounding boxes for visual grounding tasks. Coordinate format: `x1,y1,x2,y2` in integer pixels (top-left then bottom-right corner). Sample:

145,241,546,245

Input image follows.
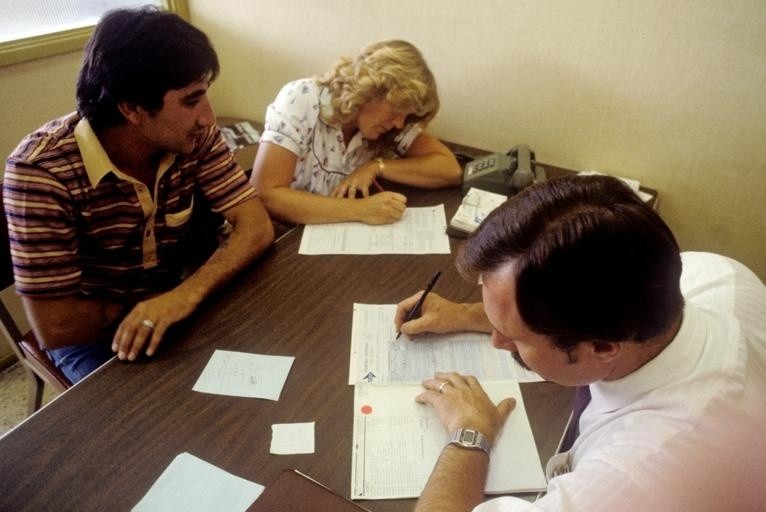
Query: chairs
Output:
0,180,73,420
231,142,295,239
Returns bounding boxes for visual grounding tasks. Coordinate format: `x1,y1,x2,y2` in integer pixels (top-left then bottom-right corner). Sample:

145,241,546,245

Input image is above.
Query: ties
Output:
557,384,592,454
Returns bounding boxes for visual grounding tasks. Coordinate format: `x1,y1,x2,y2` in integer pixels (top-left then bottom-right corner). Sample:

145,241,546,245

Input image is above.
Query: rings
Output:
142,317,154,329
438,380,449,391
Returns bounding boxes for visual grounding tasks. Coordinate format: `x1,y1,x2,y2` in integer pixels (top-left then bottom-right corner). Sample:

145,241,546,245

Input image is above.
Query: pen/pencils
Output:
371,177,383,192
395,272,440,340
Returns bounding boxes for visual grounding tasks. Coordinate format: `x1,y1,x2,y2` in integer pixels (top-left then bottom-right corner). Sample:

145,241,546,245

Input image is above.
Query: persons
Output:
249,37,464,226
393,172,766,511
2,4,278,389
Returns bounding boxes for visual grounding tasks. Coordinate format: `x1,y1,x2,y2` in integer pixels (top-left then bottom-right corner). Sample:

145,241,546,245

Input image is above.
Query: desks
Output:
0,138,659,512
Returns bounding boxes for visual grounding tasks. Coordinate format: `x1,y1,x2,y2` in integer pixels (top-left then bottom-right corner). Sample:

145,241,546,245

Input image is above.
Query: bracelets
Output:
373,156,387,178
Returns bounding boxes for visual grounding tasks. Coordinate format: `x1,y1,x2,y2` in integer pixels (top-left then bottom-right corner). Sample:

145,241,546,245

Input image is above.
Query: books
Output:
450,186,510,234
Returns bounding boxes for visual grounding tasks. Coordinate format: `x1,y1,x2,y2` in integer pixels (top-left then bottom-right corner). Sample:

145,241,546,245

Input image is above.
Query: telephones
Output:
462,145,546,195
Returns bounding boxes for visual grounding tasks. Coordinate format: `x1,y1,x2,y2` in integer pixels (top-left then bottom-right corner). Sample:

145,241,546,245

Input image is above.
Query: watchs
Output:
446,427,492,458
